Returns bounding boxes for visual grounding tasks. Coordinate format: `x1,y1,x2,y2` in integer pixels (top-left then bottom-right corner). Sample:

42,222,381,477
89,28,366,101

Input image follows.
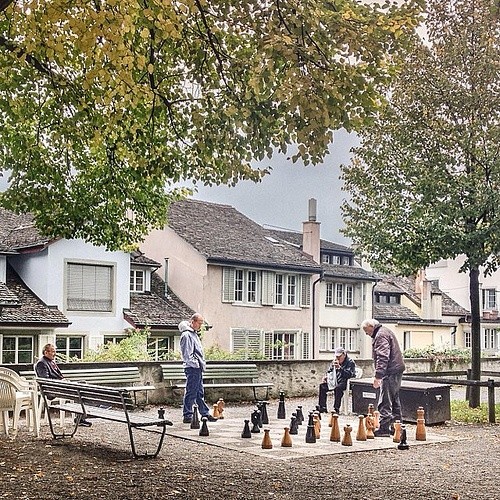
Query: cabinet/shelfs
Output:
351,378,452,426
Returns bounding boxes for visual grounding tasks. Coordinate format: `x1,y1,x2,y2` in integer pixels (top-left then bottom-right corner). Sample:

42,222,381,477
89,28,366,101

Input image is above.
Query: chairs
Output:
326,367,362,415
0,367,40,435
33,359,75,427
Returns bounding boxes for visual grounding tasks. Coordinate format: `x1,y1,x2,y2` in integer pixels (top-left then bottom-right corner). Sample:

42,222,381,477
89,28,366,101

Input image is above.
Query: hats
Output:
335,347,346,356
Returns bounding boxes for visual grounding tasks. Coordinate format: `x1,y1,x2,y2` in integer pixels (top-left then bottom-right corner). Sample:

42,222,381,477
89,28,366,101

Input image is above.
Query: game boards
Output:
131,407,461,462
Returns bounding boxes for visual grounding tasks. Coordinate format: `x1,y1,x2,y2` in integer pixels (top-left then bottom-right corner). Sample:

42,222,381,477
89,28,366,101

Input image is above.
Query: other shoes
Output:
183,415,200,424
330,409,339,414
72,418,93,427
200,414,218,422
389,429,395,435
373,428,390,437
318,407,328,413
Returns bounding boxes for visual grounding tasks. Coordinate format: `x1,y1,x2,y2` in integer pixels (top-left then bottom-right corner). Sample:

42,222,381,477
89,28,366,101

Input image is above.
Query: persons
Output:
35,344,92,427
178,313,218,423
319,347,356,413
362,318,406,436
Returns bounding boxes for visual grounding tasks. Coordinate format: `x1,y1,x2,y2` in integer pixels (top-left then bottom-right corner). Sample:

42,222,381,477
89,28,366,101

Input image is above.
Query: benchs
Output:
19,367,173,457
161,362,274,408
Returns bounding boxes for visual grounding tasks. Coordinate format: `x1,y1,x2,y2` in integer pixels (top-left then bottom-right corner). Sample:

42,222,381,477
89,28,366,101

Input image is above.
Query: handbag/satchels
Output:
326,365,338,391
